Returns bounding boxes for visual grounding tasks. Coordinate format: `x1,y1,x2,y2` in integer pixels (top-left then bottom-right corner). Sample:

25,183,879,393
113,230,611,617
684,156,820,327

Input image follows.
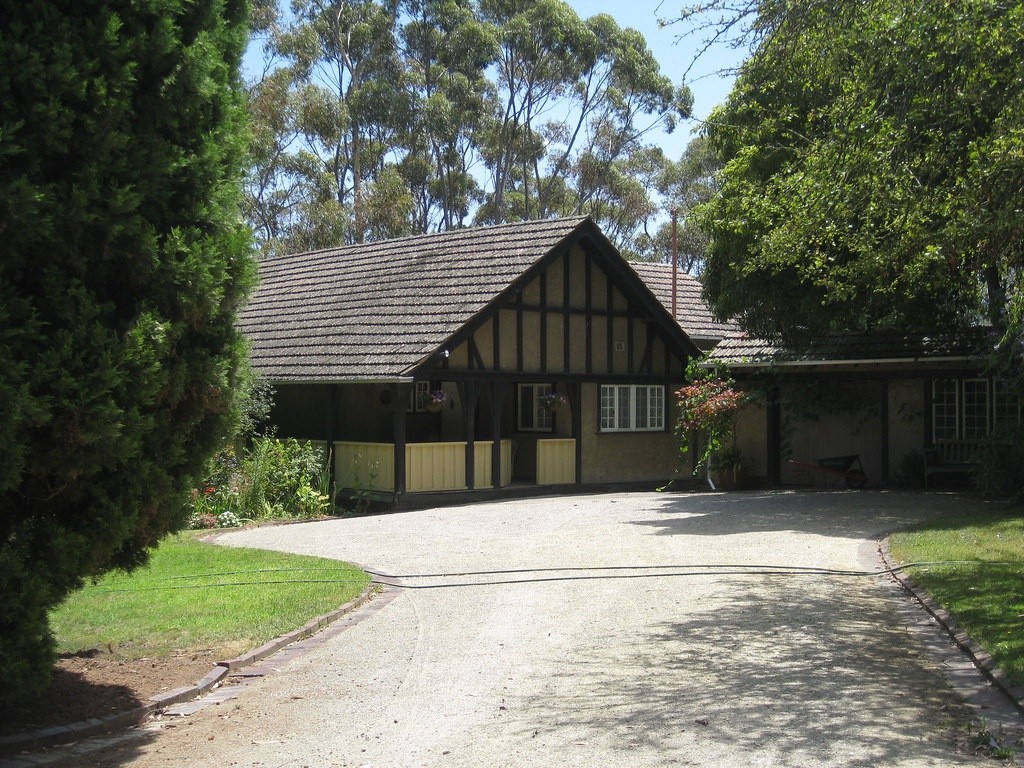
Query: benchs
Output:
924,438,1024,503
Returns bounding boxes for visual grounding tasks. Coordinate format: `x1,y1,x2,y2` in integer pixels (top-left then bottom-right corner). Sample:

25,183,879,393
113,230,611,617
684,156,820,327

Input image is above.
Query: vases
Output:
546,404,557,411
425,404,441,413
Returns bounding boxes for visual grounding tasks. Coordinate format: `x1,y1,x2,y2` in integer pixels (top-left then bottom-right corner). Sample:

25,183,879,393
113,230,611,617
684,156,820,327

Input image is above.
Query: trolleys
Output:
786,454,869,489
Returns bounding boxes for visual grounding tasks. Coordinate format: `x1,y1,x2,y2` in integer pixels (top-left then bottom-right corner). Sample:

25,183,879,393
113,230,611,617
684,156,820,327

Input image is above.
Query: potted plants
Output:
708,446,756,490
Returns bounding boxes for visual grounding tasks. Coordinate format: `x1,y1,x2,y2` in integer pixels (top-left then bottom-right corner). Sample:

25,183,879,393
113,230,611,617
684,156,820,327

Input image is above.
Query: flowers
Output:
537,392,567,406
422,390,447,406
